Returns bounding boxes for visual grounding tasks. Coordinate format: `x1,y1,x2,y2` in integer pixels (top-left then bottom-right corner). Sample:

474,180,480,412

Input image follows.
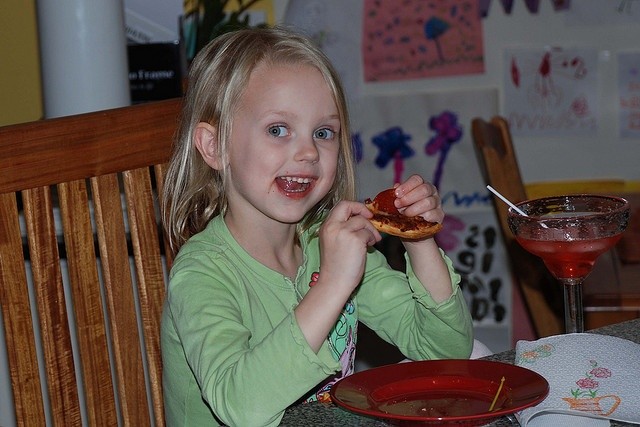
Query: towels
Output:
513,332,639,427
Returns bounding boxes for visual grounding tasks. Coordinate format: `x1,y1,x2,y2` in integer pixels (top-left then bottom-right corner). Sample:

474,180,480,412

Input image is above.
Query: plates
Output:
330,359,550,426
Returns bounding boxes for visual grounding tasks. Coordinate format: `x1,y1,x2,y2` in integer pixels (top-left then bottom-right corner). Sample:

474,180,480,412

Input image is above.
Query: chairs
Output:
0,75,222,427
470,116,640,339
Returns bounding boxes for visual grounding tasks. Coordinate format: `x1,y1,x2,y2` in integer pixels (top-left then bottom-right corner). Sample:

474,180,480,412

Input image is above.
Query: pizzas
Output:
359,187,445,241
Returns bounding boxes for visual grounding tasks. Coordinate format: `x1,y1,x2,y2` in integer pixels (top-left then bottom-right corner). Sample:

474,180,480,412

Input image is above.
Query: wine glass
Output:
507,193,629,334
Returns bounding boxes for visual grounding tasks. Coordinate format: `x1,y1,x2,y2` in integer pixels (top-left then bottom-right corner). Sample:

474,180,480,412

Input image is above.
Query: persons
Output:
159,24,475,426
